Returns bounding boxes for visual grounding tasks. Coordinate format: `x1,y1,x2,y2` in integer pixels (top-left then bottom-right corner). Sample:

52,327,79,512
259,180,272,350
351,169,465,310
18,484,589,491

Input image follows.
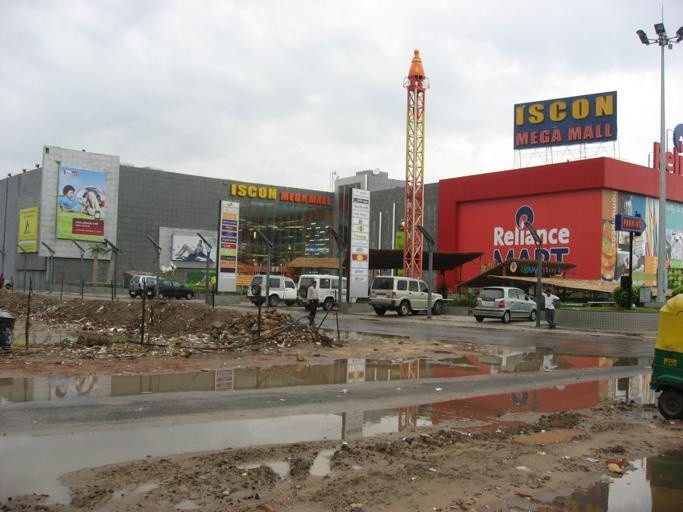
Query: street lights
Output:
415,221,436,319
254,227,276,310
323,225,348,315
69,238,85,295
15,240,55,296
523,219,543,328
637,2,683,307
102,238,120,297
196,230,212,304
145,234,162,301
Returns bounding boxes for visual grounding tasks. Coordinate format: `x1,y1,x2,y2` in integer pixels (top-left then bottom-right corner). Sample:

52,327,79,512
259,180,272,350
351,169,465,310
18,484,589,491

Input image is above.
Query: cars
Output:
145,278,195,300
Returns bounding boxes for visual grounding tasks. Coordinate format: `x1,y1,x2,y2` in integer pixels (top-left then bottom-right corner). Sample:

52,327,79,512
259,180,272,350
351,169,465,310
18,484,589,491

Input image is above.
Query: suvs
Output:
246,274,297,307
296,273,364,312
472,285,537,323
368,276,445,316
129,274,165,299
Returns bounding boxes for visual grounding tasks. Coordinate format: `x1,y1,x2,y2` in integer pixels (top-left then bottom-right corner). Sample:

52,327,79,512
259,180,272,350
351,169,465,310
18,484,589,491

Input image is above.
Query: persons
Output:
305,280,319,325
174,239,203,258
0,272,5,290
59,184,81,212
540,286,560,329
51,375,75,399
539,346,558,374
